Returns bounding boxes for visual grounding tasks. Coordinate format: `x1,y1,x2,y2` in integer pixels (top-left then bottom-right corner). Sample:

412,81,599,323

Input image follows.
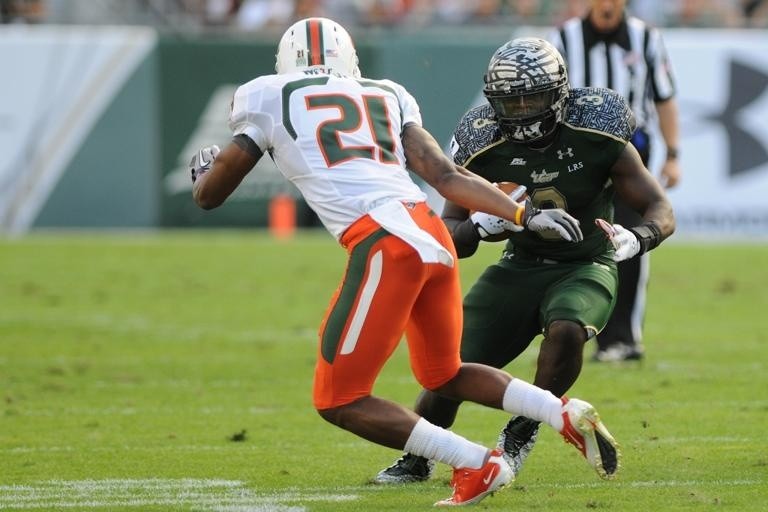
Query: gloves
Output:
595,219,641,262
189,145,220,184
523,200,583,243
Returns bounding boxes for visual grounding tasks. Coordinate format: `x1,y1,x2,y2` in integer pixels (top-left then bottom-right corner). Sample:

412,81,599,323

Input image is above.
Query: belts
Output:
503,251,558,264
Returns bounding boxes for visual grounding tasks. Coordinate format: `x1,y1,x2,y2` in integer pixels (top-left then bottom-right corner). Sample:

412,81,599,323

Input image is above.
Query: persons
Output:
548,0,684,363
363,34,676,486
185,15,622,508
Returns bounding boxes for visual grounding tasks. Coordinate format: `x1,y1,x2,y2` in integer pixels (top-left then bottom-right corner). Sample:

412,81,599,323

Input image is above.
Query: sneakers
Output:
434,449,515,508
596,341,642,362
377,454,434,483
497,416,542,476
560,397,621,480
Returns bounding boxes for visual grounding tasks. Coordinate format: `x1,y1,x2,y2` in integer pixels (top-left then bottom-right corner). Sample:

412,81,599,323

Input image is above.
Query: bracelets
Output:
514,205,525,226
665,148,679,161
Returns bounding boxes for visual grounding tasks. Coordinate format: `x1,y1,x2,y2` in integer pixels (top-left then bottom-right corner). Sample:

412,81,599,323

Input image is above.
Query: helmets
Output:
275,16,361,78
483,38,571,144
